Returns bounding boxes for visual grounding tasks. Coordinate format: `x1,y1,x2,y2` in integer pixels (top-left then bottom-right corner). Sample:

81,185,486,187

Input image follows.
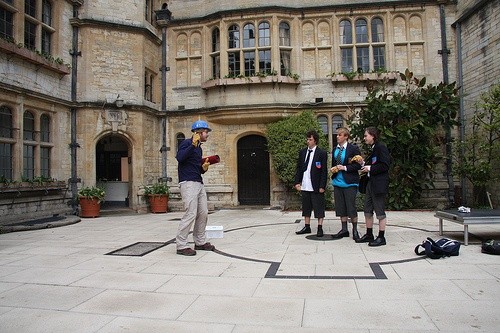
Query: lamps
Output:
115,94,123,107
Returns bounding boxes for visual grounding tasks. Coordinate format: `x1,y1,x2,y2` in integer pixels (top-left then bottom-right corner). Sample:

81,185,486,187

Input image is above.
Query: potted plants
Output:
77,186,105,218
143,179,170,213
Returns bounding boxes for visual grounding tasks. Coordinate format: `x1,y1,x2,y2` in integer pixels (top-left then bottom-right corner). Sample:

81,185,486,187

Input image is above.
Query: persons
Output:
329,127,365,241
174,120,215,256
294,130,328,237
355,127,390,247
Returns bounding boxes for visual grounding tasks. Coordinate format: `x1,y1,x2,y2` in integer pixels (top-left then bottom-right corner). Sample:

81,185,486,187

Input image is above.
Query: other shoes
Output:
194,243,215,250
177,248,196,256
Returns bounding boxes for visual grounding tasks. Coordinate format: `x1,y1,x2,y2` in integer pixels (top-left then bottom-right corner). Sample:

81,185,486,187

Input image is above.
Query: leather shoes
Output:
331,230,349,239
295,227,311,234
368,236,386,246
353,230,359,240
317,229,324,237
355,234,374,243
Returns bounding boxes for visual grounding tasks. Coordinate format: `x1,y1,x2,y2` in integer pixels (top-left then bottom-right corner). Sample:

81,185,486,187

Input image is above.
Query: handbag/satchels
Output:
359,175,368,193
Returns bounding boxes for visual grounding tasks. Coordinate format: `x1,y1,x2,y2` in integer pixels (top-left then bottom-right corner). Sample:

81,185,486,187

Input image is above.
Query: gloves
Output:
201,157,211,171
192,134,201,148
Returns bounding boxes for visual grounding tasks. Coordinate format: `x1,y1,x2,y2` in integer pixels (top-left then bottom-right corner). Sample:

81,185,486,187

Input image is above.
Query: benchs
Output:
434,209,500,245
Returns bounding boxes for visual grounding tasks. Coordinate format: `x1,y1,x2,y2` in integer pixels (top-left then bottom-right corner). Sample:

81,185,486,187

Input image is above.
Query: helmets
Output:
191,121,211,133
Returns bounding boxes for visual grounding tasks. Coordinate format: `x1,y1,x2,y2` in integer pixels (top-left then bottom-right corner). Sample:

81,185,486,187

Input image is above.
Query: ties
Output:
336,147,345,166
301,150,313,172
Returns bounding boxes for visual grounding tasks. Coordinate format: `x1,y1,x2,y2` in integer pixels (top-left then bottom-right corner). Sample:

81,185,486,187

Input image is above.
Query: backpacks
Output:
414,237,461,259
481,240,500,255
